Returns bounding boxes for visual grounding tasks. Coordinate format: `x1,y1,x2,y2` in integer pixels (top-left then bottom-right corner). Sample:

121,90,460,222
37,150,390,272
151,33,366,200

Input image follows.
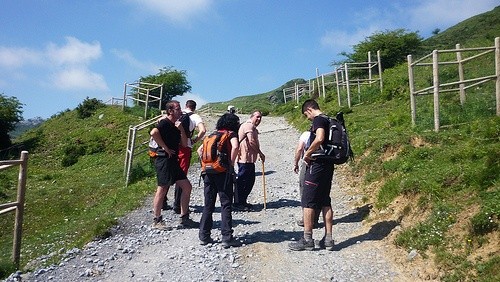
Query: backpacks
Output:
317,115,349,165
202,129,232,174
179,112,195,137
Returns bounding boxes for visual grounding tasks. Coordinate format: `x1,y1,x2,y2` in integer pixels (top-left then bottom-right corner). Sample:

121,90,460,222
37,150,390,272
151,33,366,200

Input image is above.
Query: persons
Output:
234,109,265,211
161,100,206,213
196,113,240,247
293,130,320,227
226,105,238,119
287,99,334,252
149,100,199,230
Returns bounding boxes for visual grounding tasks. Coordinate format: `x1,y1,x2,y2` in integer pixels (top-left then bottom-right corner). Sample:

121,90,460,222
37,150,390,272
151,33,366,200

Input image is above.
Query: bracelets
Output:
193,139,196,143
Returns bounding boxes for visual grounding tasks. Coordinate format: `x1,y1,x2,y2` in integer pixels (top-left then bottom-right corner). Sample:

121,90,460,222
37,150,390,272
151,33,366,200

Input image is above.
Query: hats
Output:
227,106,235,111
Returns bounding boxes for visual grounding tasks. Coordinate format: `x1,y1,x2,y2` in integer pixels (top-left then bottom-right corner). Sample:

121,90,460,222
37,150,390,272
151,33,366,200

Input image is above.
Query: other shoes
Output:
200,238,213,245
289,239,315,251
152,221,172,231
316,238,333,250
236,206,254,212
222,239,241,246
178,217,200,228
299,221,318,227
163,206,171,210
